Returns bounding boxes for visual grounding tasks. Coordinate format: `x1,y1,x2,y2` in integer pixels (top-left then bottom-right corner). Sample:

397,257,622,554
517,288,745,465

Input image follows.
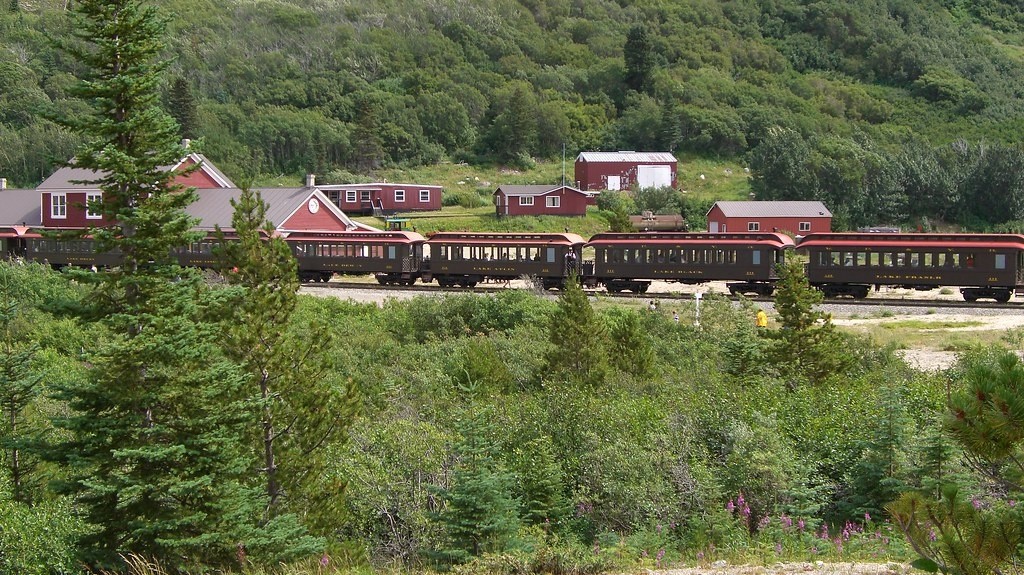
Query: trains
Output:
1,221,1024,302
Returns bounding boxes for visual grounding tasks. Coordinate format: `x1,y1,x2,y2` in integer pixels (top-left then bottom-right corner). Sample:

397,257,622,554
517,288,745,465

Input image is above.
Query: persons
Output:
613,251,687,263
44,258,50,265
673,311,680,322
472,252,540,262
231,264,237,273
6,251,25,266
68,262,74,281
756,307,767,334
565,248,576,276
832,253,974,268
783,324,789,338
90,263,97,273
647,301,661,311
